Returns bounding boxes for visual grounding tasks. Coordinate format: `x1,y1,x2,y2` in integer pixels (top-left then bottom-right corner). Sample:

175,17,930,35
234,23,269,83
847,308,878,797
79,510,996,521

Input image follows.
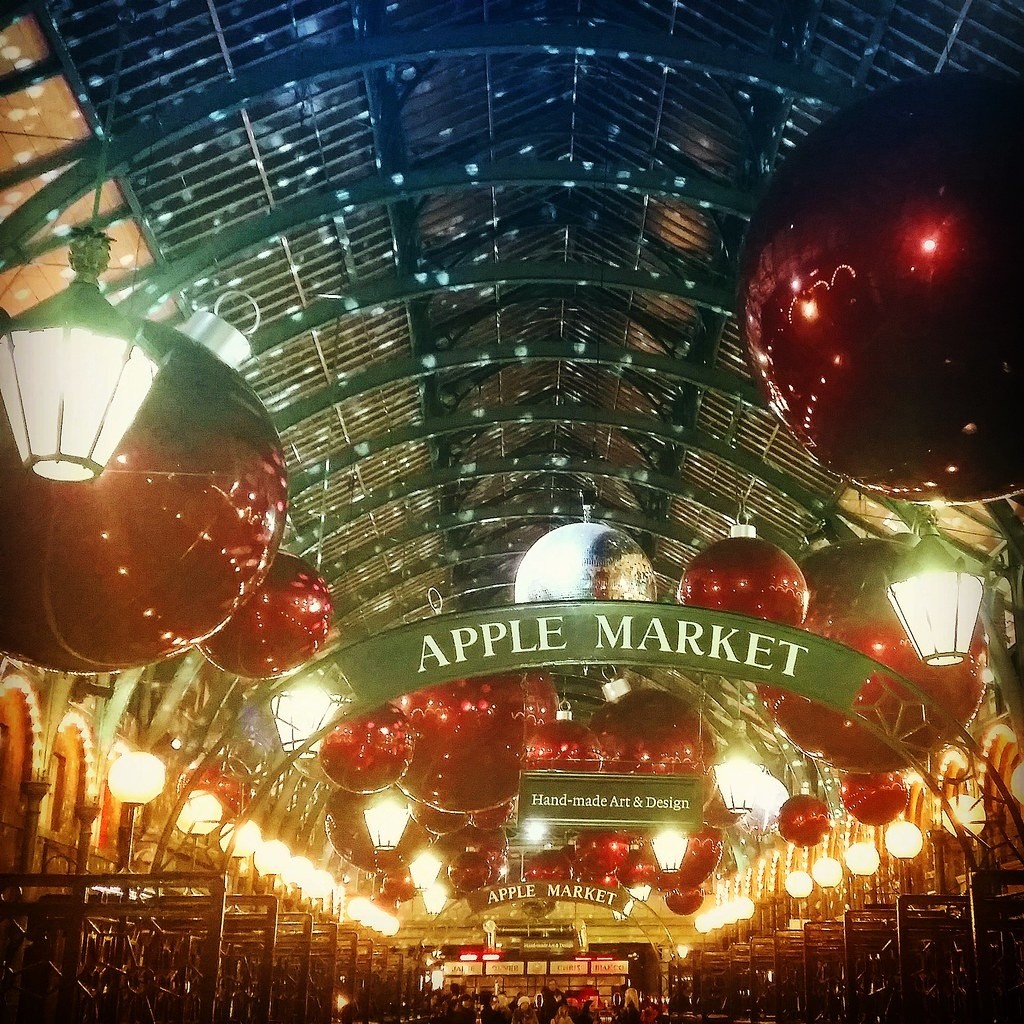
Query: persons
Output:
668,982,690,1014
479,988,527,1024
609,1001,643,1024
430,983,477,1024
536,979,567,1024
551,1005,574,1024
574,1000,598,1024
510,996,540,1024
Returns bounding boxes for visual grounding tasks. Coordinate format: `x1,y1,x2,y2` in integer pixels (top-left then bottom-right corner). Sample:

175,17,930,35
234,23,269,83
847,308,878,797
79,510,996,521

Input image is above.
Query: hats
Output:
517,997,530,1008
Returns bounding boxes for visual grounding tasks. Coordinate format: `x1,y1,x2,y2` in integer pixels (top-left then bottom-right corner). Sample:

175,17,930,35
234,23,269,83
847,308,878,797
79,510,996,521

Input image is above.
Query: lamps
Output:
650,831,689,874
713,678,768,815
270,234,350,758
885,509,984,666
0,10,175,486
364,788,414,852
